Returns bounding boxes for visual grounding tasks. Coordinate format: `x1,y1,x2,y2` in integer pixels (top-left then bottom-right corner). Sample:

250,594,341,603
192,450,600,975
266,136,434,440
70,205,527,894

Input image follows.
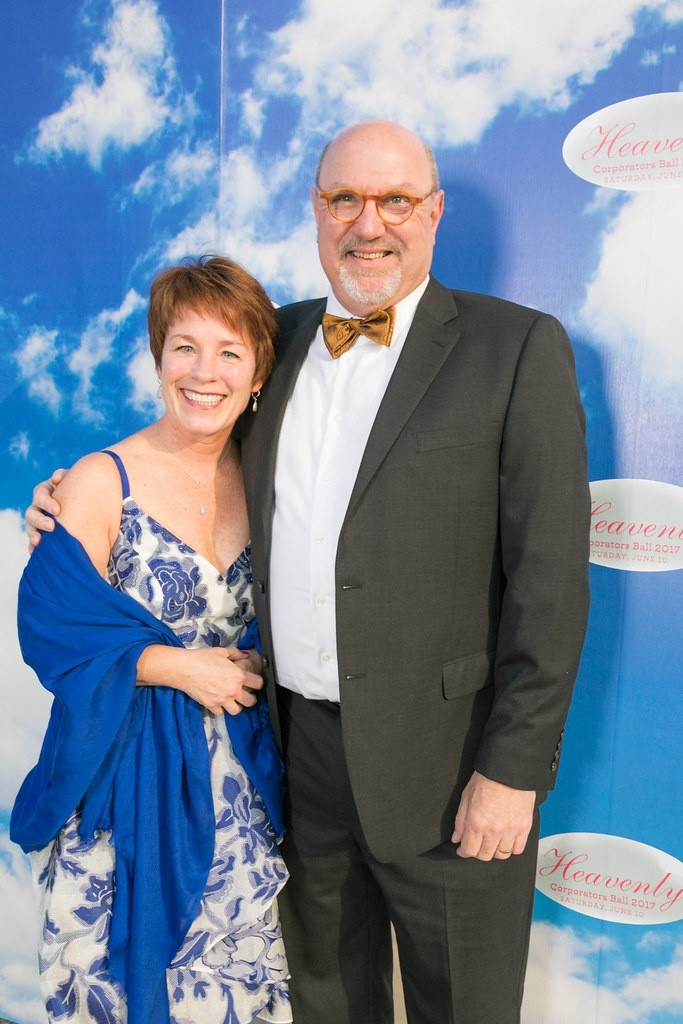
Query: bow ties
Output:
322,305,396,360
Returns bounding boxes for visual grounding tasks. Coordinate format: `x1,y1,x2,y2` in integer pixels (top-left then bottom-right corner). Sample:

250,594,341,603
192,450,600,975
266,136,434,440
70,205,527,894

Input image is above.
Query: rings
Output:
497,847,512,854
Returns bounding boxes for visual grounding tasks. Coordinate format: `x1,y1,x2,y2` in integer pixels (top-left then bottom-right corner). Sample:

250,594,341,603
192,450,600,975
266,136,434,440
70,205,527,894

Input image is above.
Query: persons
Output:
8,249,295,1023
24,121,593,1024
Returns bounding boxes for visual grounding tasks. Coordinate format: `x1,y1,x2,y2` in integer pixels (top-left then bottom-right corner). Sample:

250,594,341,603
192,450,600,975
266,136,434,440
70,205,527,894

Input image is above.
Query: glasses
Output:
314,184,434,226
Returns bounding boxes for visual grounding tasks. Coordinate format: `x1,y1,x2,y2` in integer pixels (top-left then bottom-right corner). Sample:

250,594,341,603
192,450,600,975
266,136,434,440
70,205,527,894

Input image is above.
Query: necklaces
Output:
157,418,232,515
